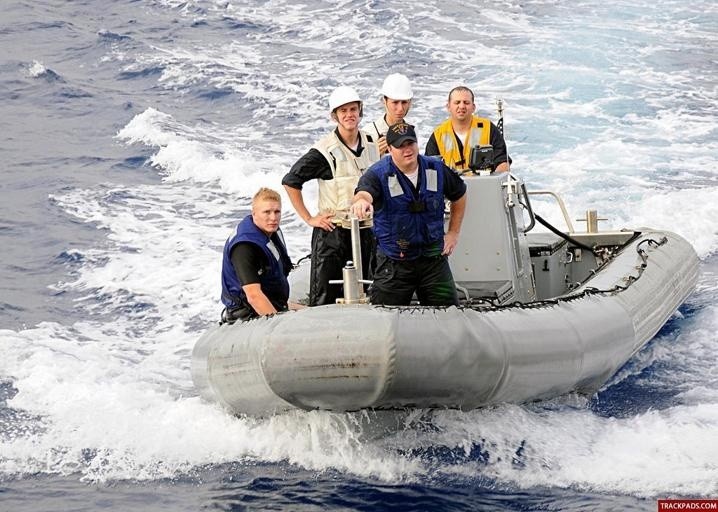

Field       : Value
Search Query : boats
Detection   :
[188,226,705,439]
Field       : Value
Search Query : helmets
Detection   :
[327,86,364,113]
[379,72,415,100]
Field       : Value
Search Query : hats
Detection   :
[384,122,419,149]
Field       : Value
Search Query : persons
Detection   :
[219,187,310,322]
[359,72,419,158]
[282,84,383,304]
[424,86,513,174]
[349,122,466,307]
